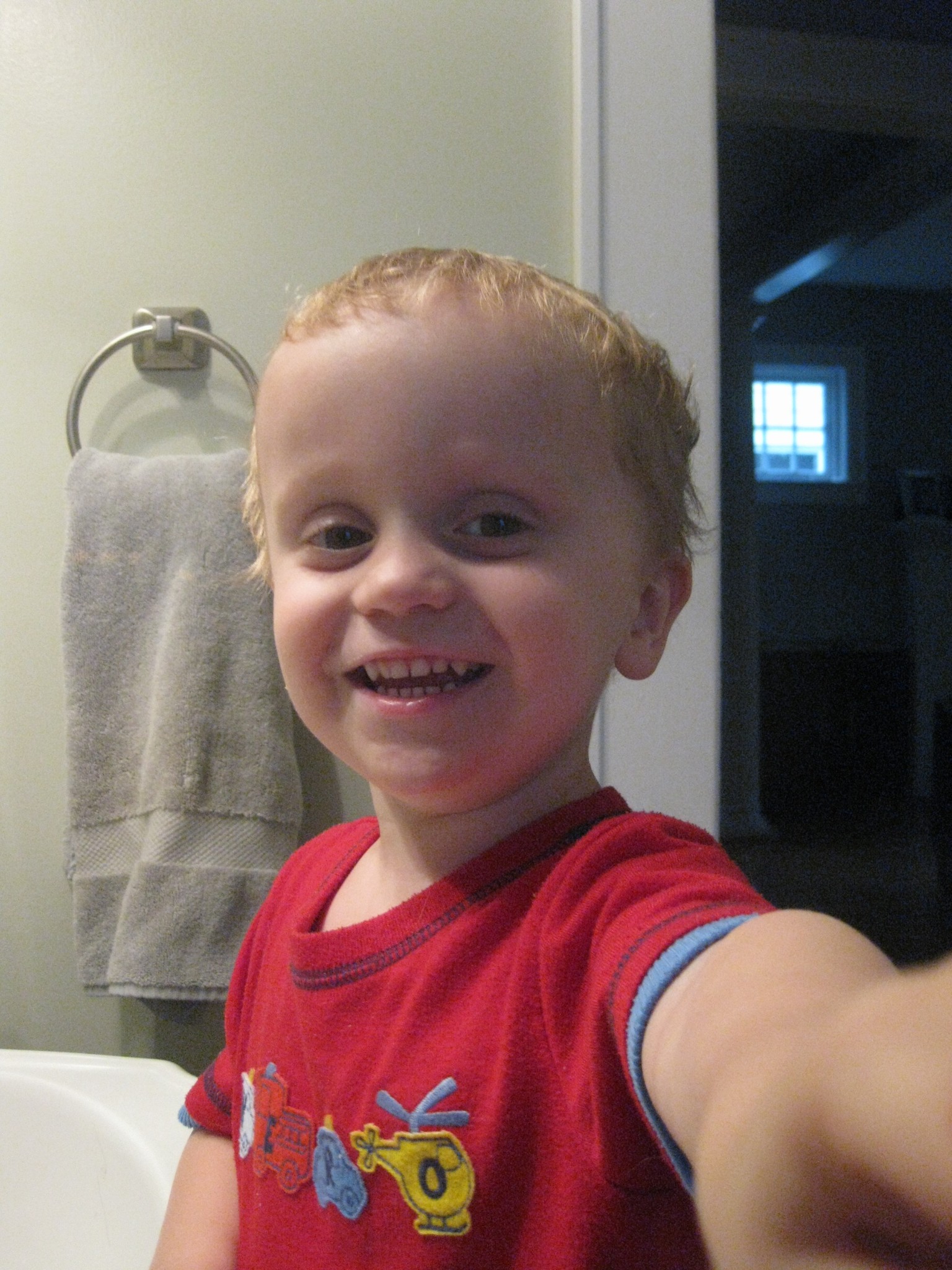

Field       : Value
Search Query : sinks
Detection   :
[3,1047,210,1264]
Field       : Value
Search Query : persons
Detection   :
[147,247,952,1270]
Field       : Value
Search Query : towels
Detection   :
[59,446,312,1008]
